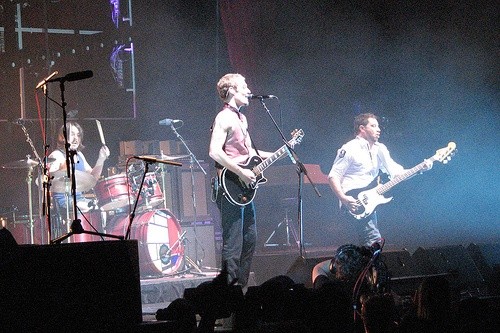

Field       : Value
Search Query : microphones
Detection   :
[49,70,93,82]
[135,155,183,166]
[159,118,179,125]
[35,71,58,89]
[248,94,274,99]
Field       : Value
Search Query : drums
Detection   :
[49,209,107,244]
[106,208,185,275]
[129,172,164,209]
[92,173,137,211]
[1,215,50,245]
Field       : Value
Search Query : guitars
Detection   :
[220,128,306,206]
[344,140,459,220]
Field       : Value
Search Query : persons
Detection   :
[312,244,500,333]
[36,121,110,238]
[327,112,433,248]
[209,74,294,289]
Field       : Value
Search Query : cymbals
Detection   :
[156,153,191,161]
[0,157,56,168]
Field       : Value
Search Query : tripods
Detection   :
[168,125,224,274]
[48,82,124,244]
[266,198,299,249]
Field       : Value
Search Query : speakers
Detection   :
[286,256,334,288]
[164,163,210,220]
[409,246,484,290]
[178,220,217,272]
[468,242,500,284]
[376,249,421,294]
